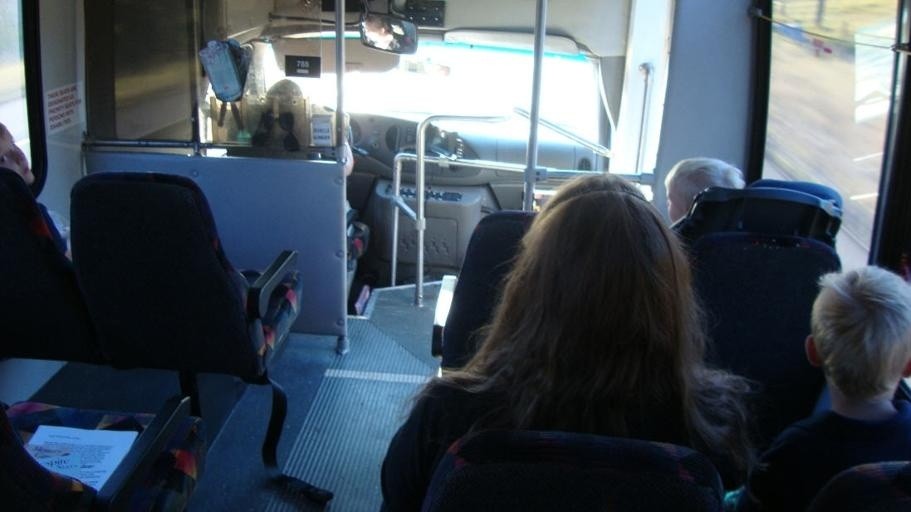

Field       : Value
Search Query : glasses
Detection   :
[276,109,301,154]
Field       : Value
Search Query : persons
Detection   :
[664,156,747,227]
[747,264,911,512]
[216,78,355,178]
[362,18,414,52]
[376,167,746,511]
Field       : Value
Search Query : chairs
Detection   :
[0,384,204,512]
[70,172,310,418]
[228,98,369,273]
[0,167,75,361]
